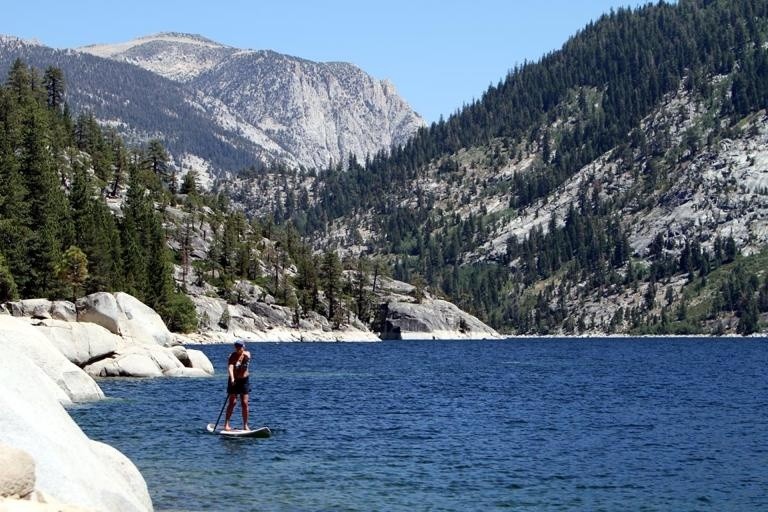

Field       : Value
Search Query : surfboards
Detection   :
[205,423,270,437]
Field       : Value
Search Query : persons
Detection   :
[224,339,251,431]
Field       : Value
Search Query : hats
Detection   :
[235,341,245,347]
